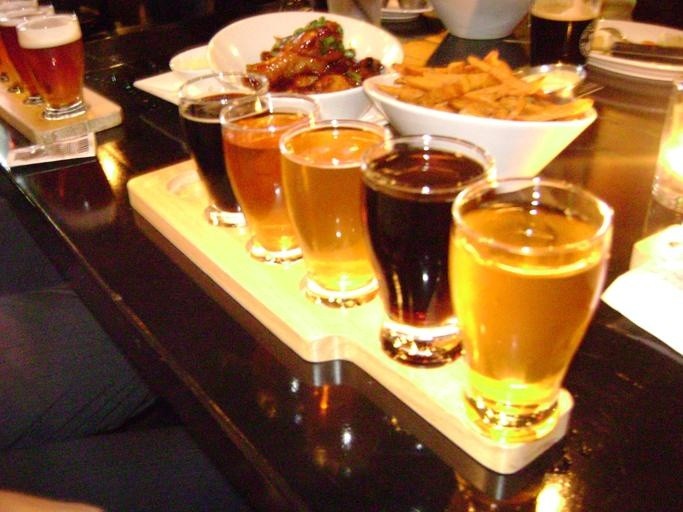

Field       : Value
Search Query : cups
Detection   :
[0,0,86,121]
[362,132,495,368]
[169,45,209,82]
[527,0,601,70]
[650,80,683,215]
[215,93,319,265]
[176,71,271,228]
[445,170,615,446]
[277,118,395,306]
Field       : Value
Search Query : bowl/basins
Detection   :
[208,12,405,124]
[362,71,600,185]
[431,0,530,40]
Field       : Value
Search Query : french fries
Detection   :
[376,48,595,122]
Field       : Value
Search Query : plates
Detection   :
[380,5,434,23]
[584,21,683,85]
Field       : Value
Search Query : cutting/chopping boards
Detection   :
[126,155,573,479]
[0,78,125,146]
